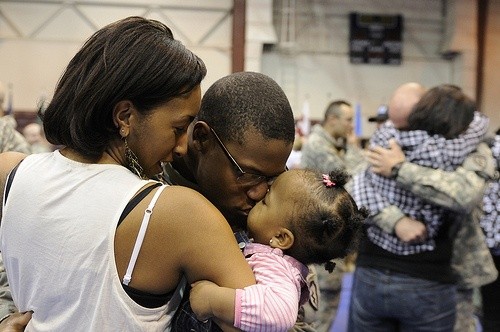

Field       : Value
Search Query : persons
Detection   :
[300,100,365,332]
[0,71,298,332]
[0,90,53,154]
[186,167,370,332]
[0,13,258,332]
[344,81,498,332]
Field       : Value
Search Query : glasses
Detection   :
[210,128,290,187]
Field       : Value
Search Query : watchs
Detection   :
[390,161,405,179]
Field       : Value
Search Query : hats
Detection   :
[368,105,389,122]
